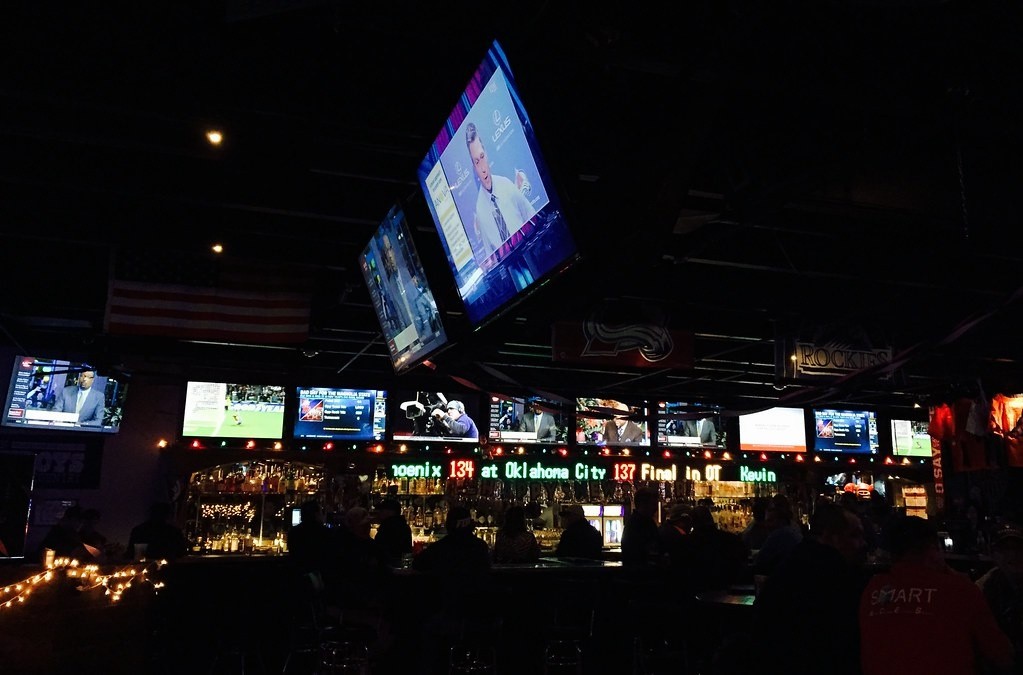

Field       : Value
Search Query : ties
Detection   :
[618,428,623,440]
[697,422,702,436]
[491,195,511,240]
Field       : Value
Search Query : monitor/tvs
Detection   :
[888,418,933,460]
[293,384,389,442]
[393,388,481,447]
[0,352,135,437]
[416,38,582,333]
[573,394,653,450]
[654,396,728,451]
[177,379,288,442]
[360,201,461,377]
[736,405,809,454]
[812,405,883,456]
[487,392,571,447]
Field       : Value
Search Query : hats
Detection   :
[989,521,1023,546]
[558,505,584,517]
[447,400,465,415]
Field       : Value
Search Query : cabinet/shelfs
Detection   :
[186,490,446,559]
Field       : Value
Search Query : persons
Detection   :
[494,507,540,563]
[431,401,478,438]
[465,123,535,254]
[35,509,185,561]
[255,495,338,553]
[382,234,433,342]
[52,370,105,425]
[937,501,998,560]
[860,516,1013,675]
[750,504,872,675]
[225,384,285,425]
[375,500,412,568]
[684,411,716,445]
[413,506,490,628]
[556,506,601,559]
[977,521,1023,675]
[622,487,745,591]
[313,508,391,656]
[520,407,556,441]
[605,404,642,442]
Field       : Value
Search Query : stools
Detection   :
[144,567,758,675]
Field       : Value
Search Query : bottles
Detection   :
[184,461,931,557]
